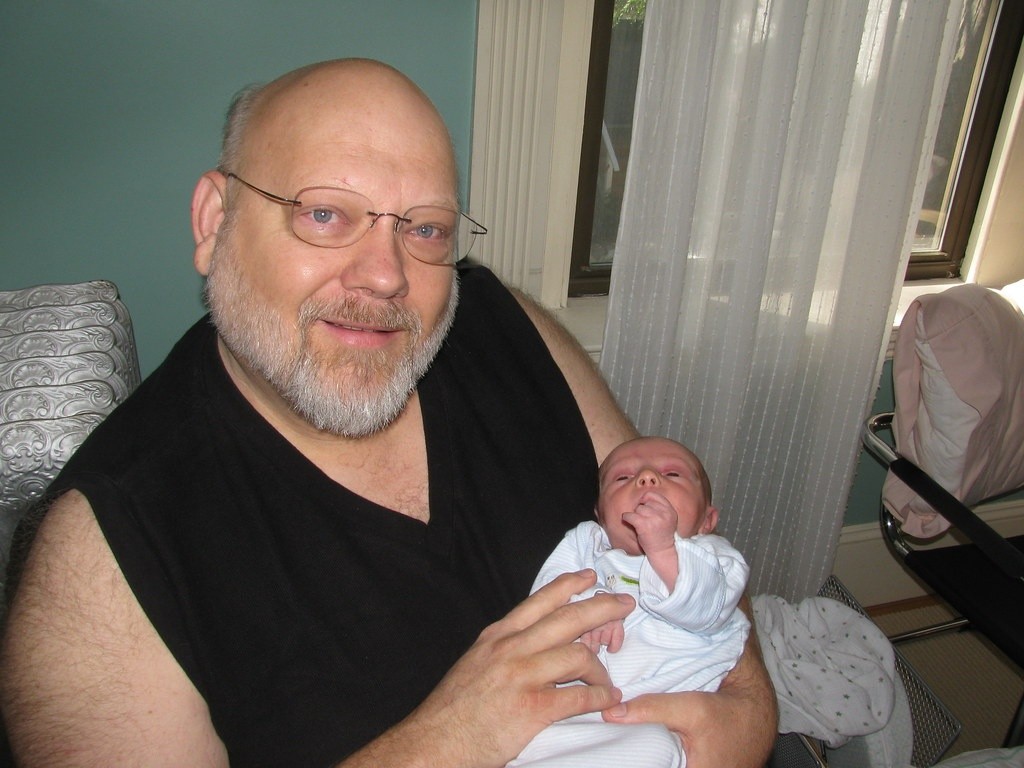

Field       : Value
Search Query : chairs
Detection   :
[856,410,1022,768]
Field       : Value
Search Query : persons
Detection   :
[0,57,780,767]
[507,435,752,768]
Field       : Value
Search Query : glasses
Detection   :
[216,166,488,267]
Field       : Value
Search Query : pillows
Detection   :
[880,288,1024,545]
